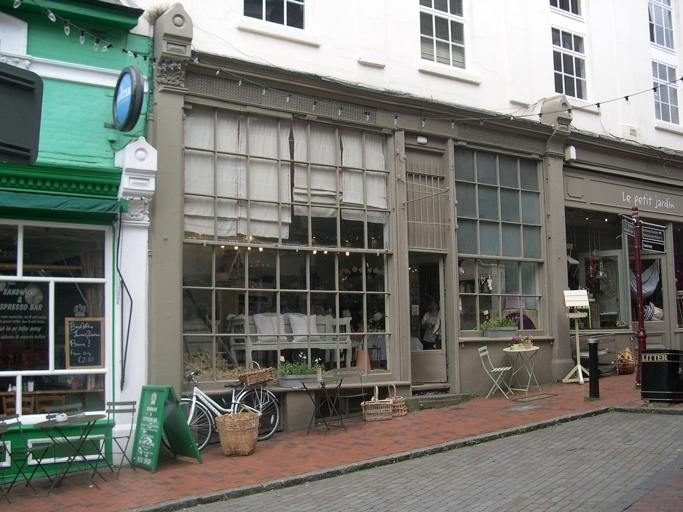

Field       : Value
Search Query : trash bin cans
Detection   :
[640,349,683,403]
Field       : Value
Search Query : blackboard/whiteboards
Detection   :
[0,279,48,348]
[64,317,104,369]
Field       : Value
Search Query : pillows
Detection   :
[253,313,288,344]
[287,314,322,342]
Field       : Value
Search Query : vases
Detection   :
[511,344,520,350]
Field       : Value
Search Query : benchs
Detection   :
[226,313,353,369]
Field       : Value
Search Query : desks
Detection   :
[503,346,541,398]
[299,377,348,435]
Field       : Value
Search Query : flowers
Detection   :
[512,335,522,344]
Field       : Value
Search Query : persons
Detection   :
[420,301,439,350]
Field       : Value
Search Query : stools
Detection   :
[368,347,382,369]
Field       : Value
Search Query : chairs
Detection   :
[334,367,368,429]
[478,346,514,400]
[0,393,136,505]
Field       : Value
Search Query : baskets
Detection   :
[613,348,637,376]
[237,359,276,387]
[385,382,408,417]
[215,411,260,457]
[358,385,393,421]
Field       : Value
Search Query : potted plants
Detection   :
[524,333,534,349]
[277,363,317,387]
[481,316,519,338]
